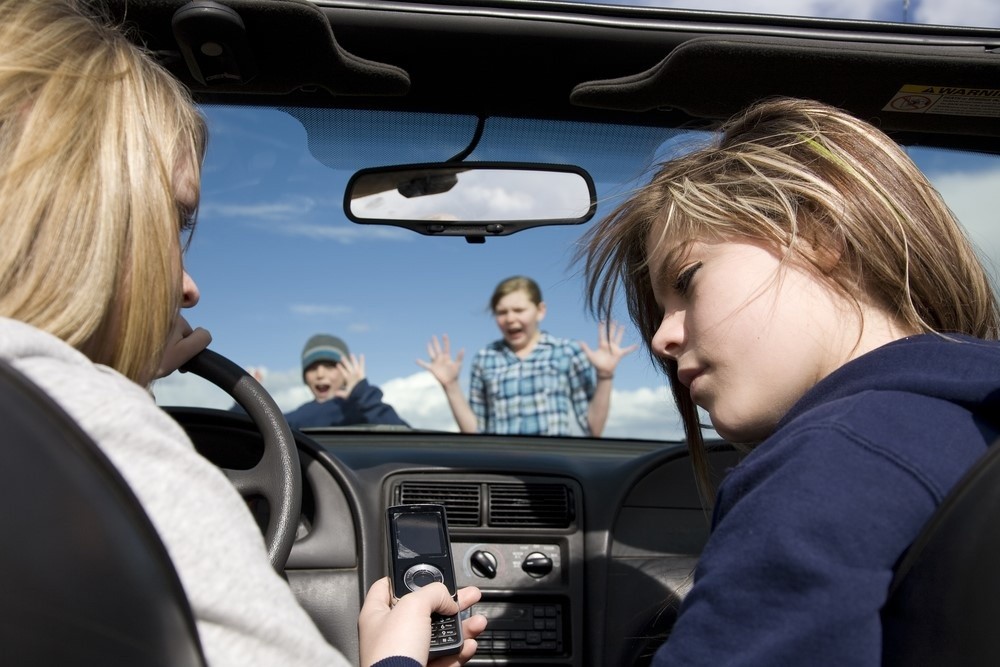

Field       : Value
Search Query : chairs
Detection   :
[0,355,208,667]
[880,440,1000,667]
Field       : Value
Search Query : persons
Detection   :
[359,101,1000,667]
[0,0,353,667]
[415,276,636,437]
[226,334,411,427]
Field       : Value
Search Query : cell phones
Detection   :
[386,502,464,656]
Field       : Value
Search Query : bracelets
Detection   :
[596,374,614,379]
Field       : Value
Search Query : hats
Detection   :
[301,334,350,384]
